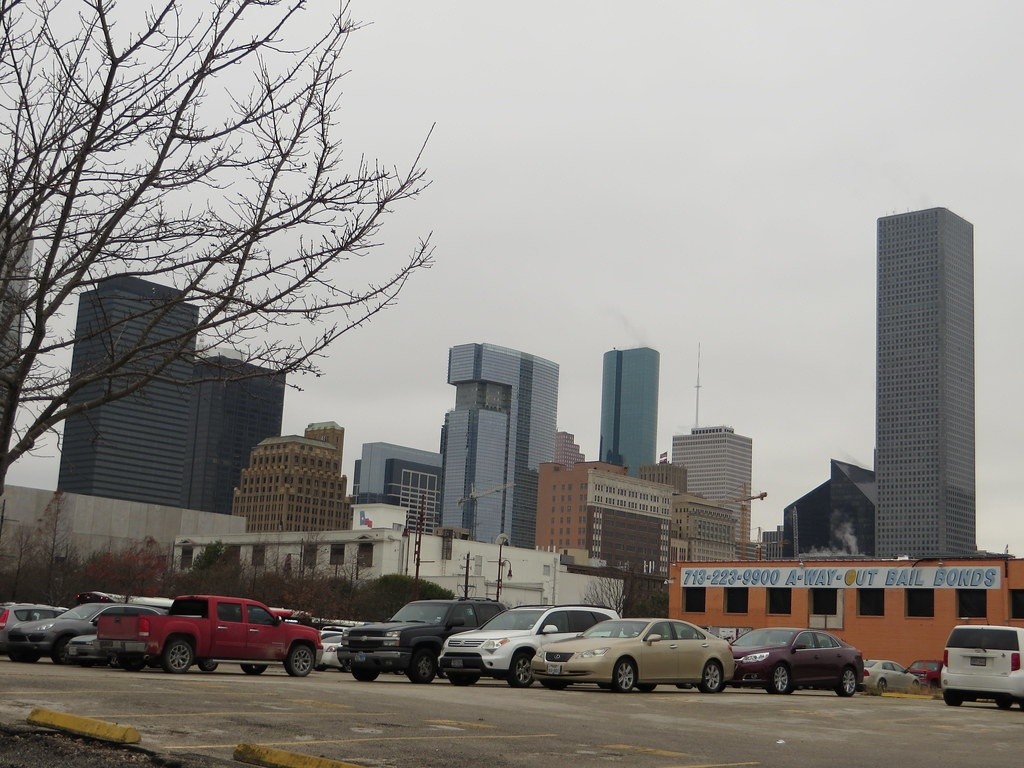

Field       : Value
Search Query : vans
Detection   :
[940,625,1023,712]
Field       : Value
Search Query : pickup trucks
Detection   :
[97,595,325,678]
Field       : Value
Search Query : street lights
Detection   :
[496,536,513,602]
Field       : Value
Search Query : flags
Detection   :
[660,452,667,458]
[365,519,372,529]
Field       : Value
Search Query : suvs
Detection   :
[438,604,625,688]
[337,598,515,684]
[0,602,81,664]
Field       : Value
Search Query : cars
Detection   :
[528,618,736,695]
[906,659,946,691]
[730,626,865,698]
[5,602,170,664]
[858,659,922,696]
[250,605,349,673]
[64,634,121,669]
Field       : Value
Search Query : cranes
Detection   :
[716,484,767,561]
[747,527,792,562]
[458,480,517,541]
